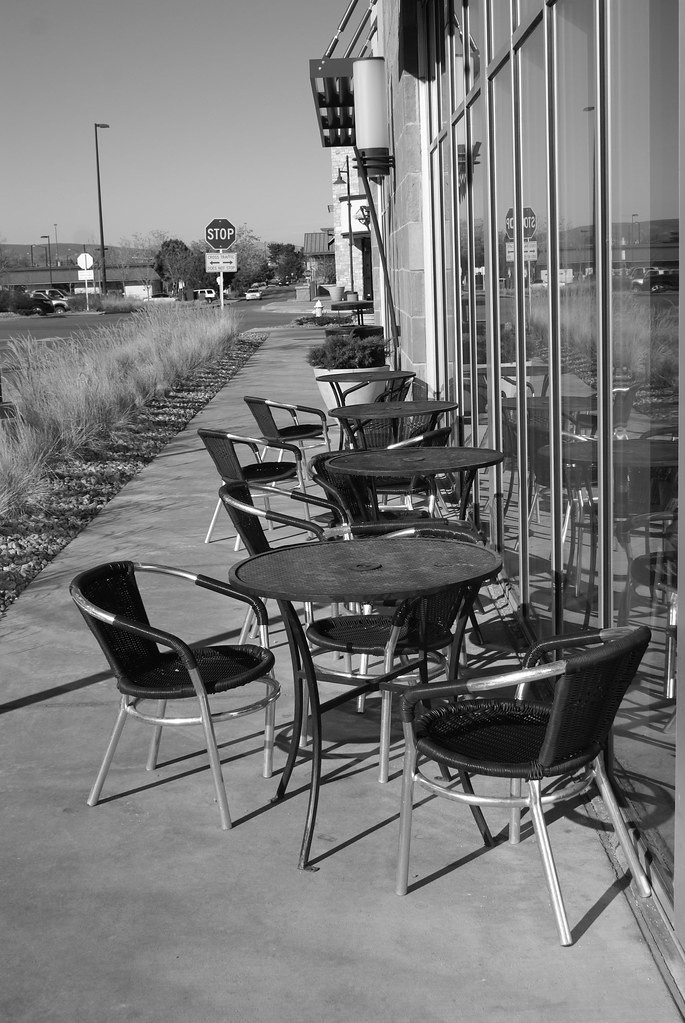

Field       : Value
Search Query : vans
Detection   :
[193,288,217,304]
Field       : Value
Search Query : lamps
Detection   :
[351,56,397,183]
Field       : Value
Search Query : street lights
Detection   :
[95,123,110,296]
[41,236,52,289]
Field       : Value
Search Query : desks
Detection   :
[326,445,505,531]
[227,535,506,876]
[331,301,374,328]
[327,399,458,453]
[316,370,418,452]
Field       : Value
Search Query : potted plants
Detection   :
[305,335,394,427]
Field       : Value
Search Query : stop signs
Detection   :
[205,218,236,250]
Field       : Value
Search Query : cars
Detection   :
[29,289,73,314]
[143,293,179,302]
[245,287,262,301]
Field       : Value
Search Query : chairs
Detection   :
[395,623,655,948]
[68,557,282,834]
[195,427,312,531]
[378,425,453,519]
[243,396,332,489]
[217,478,356,649]
[303,521,489,714]
[507,336,677,605]
